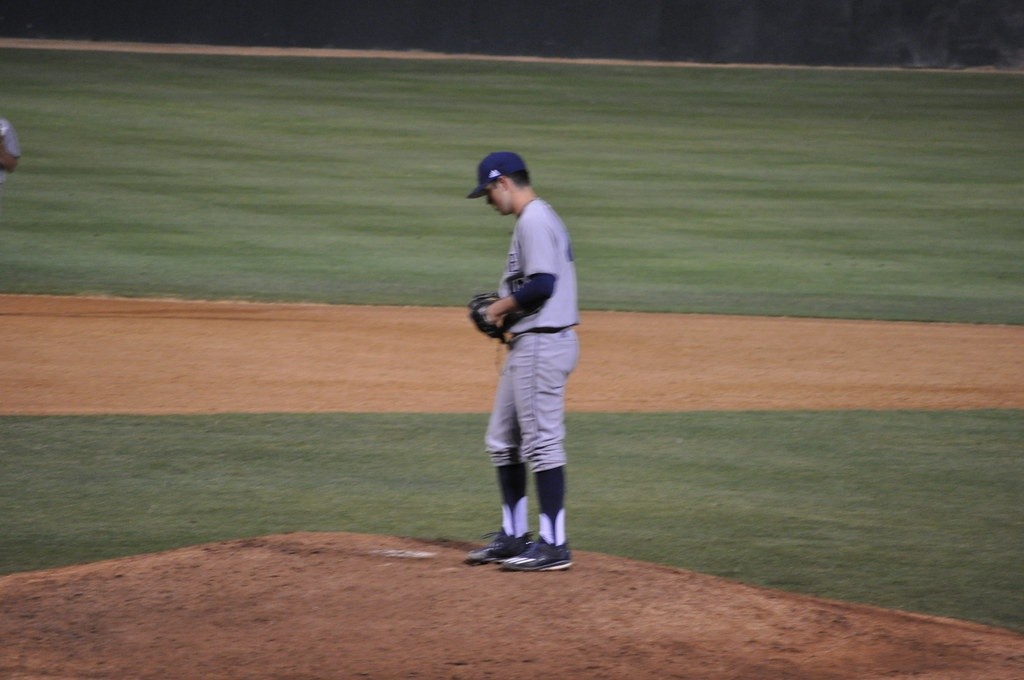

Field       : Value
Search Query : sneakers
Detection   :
[503,537,573,571]
[463,527,534,566]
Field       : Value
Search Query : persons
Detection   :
[465,151,584,574]
[0,116,22,184]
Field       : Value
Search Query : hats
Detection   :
[465,151,527,199]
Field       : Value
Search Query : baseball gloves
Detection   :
[466,292,510,338]
